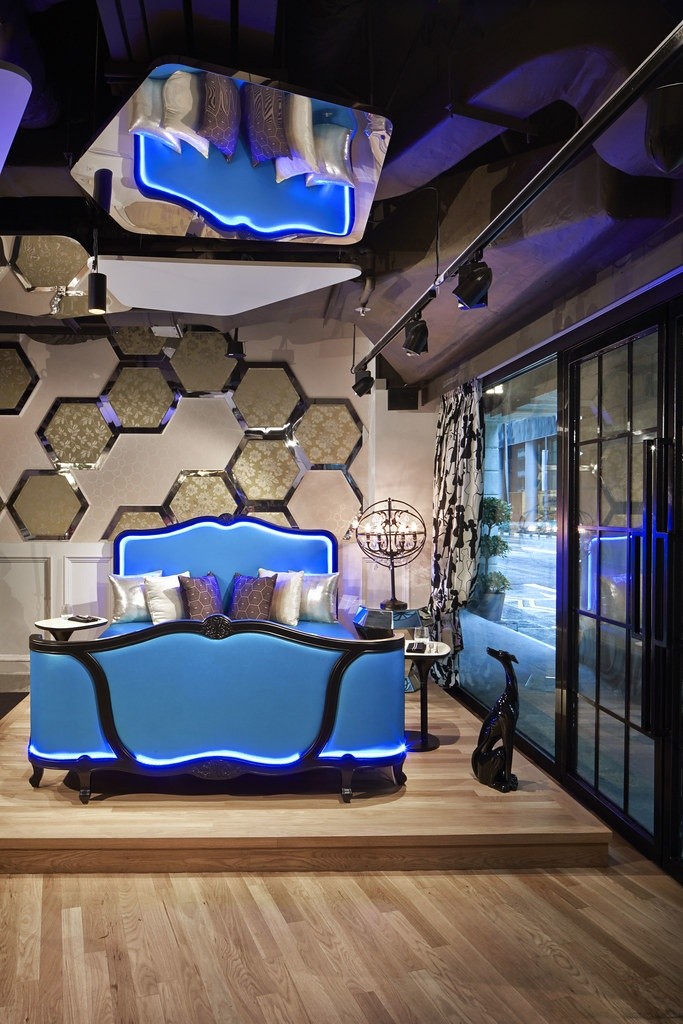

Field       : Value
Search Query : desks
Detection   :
[34,614,108,642]
[405,638,451,752]
[352,605,433,692]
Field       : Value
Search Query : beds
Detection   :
[26,513,407,803]
[134,63,357,241]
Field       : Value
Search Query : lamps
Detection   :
[87,261,107,315]
[644,64,683,173]
[226,341,246,358]
[353,364,375,397]
[355,498,427,610]
[452,249,492,310]
[403,312,429,357]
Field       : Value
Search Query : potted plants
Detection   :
[468,496,514,621]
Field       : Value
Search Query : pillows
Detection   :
[197,71,241,164]
[238,82,295,167]
[128,77,183,155]
[276,94,321,184]
[178,570,224,620]
[257,568,304,626]
[227,572,279,620]
[107,570,163,623]
[288,569,340,625]
[144,571,191,626]
[160,71,211,159]
[306,123,355,189]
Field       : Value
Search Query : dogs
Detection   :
[471,647,519,793]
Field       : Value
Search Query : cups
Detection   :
[414,627,429,644]
